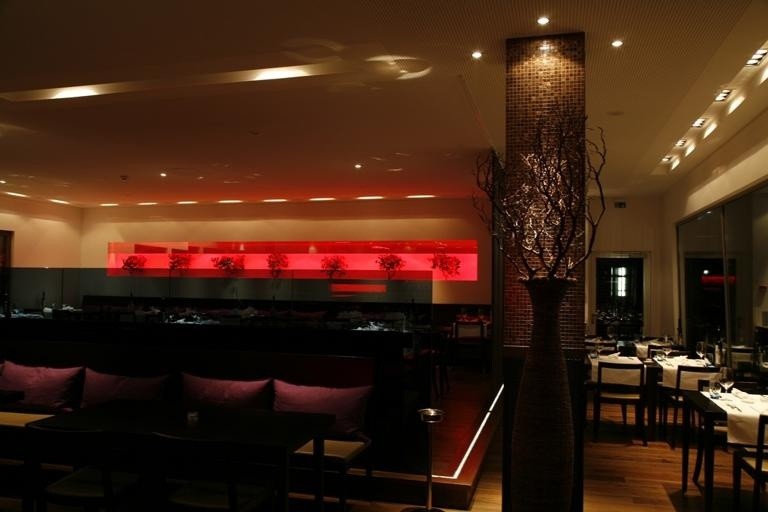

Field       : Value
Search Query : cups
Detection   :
[708,379,721,395]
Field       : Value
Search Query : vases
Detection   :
[508,277,576,511]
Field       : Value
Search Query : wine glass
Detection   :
[718,366,735,396]
[661,342,673,359]
[695,341,705,360]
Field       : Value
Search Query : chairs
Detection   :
[584,322,768,512]
